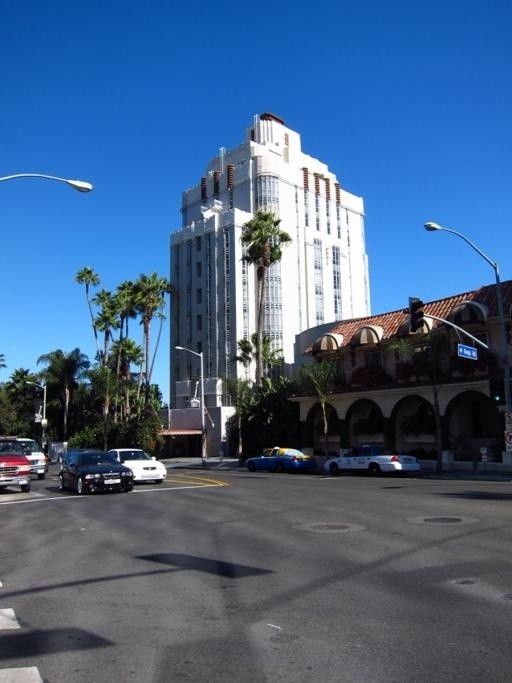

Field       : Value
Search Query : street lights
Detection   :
[424,222,512,464]
[26,382,46,448]
[0,174,93,192]
[175,346,207,467]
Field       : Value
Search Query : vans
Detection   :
[0,436,47,492]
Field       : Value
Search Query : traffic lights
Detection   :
[488,367,506,405]
[409,297,424,334]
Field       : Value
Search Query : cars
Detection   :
[58,449,166,495]
[324,445,421,476]
[245,447,317,472]
